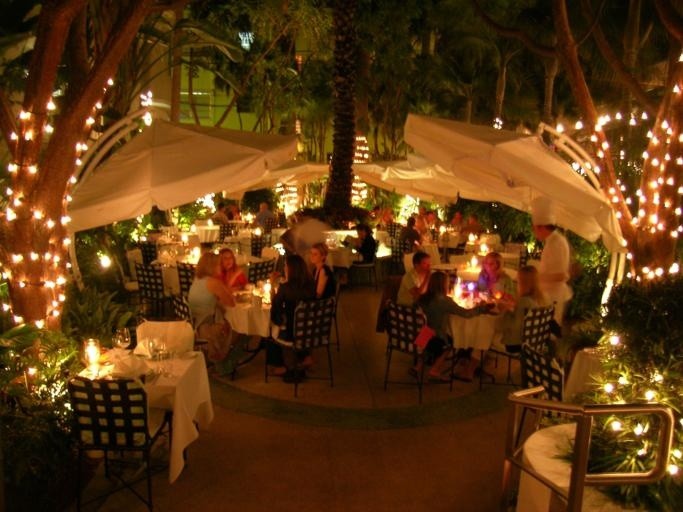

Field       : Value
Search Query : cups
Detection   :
[83,339,103,376]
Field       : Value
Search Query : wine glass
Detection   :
[450,277,504,311]
[244,280,278,304]
[146,337,173,377]
[326,234,341,248]
[112,327,132,361]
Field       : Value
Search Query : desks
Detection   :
[563,345,615,400]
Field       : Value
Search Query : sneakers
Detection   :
[408,367,474,385]
[282,362,314,384]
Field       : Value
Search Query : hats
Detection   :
[529,196,557,225]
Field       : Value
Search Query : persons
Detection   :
[531,198,574,342]
[188,253,264,364]
[385,202,545,382]
[266,253,317,374]
[310,242,334,301]
[218,248,249,295]
[212,202,276,232]
[347,223,376,263]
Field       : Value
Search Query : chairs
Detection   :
[72,205,379,399]
[377,223,565,450]
[67,375,172,511]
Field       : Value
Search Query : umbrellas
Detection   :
[221,158,331,195]
[59,104,298,295]
[403,113,630,326]
[352,152,502,209]
